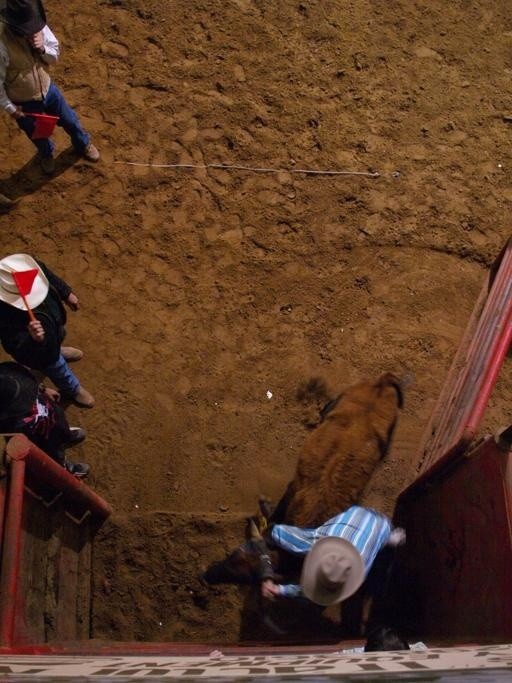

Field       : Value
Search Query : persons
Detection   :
[0,0,101,175]
[1,253,98,409]
[263,505,408,608]
[0,359,93,480]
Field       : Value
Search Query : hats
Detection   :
[1,1,47,35]
[299,536,364,606]
[1,362,37,419]
[0,254,50,311]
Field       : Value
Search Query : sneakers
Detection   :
[65,463,89,478]
[74,388,96,408]
[41,161,55,174]
[60,347,83,361]
[68,427,85,442]
[85,145,100,161]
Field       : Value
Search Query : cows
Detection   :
[251,369,407,568]
